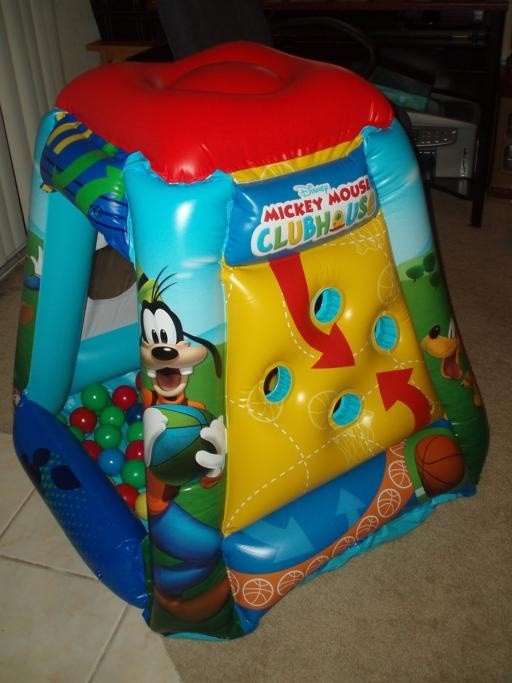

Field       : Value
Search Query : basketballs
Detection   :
[415,435,464,496]
[150,405,218,487]
